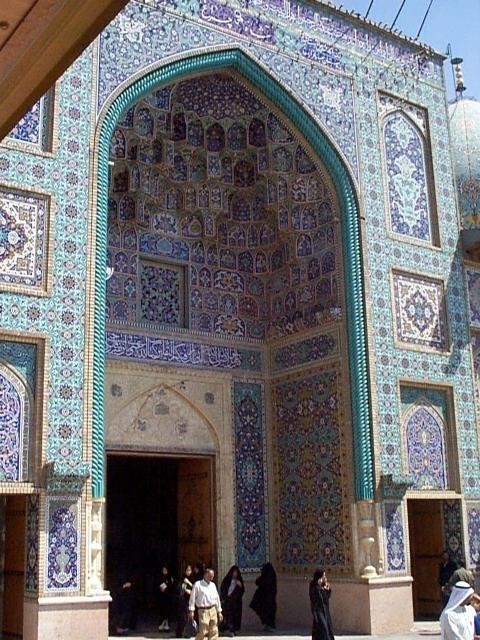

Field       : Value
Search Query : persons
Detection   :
[309,568,335,640]
[110,565,245,640]
[439,551,480,640]
[250,563,277,632]
